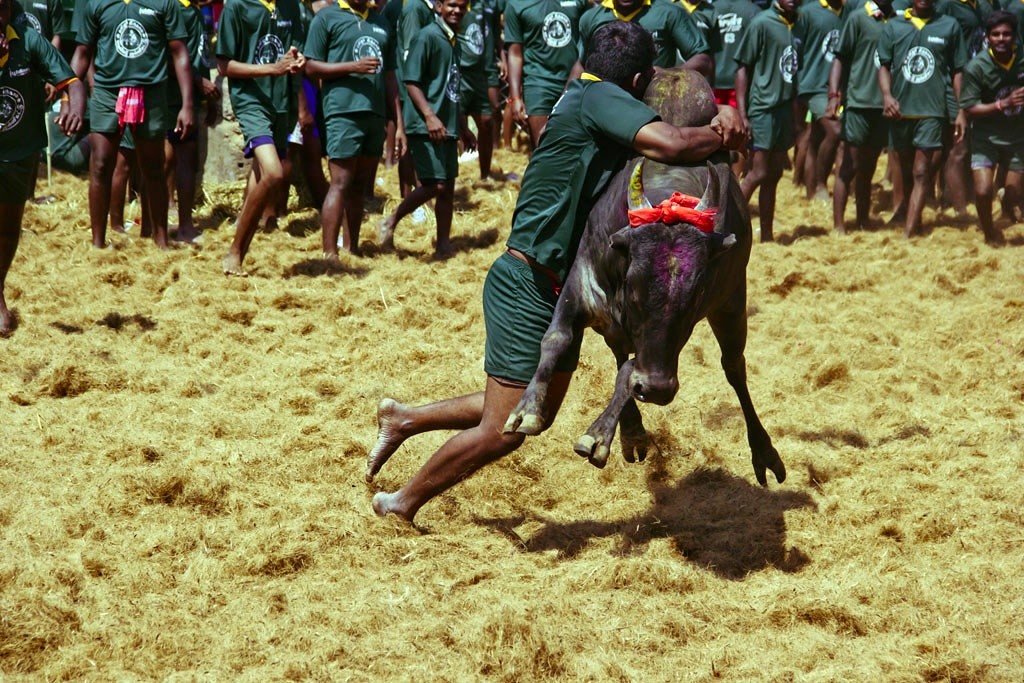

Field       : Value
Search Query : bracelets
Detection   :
[828,92,838,100]
[996,100,1002,112]
[510,96,521,103]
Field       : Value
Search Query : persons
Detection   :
[366,21,749,523]
[0,0,1024,332]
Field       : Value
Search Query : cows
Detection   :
[504,69,788,489]
